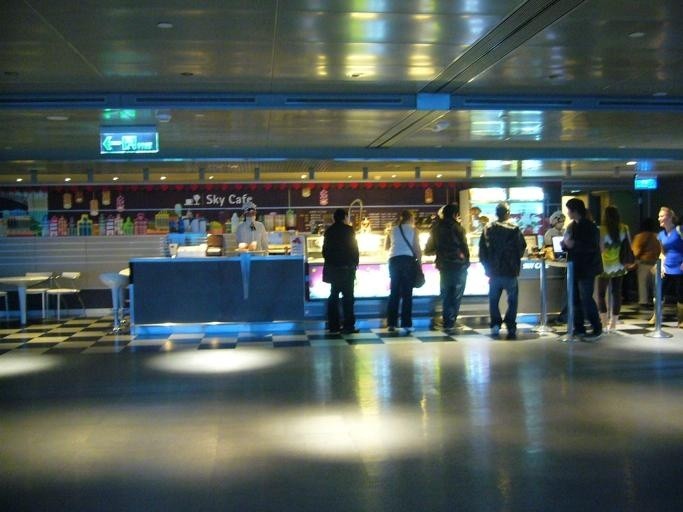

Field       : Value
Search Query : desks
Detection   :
[1,277,48,328]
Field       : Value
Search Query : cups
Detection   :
[168,244,178,258]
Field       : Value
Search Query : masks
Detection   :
[556,223,564,230]
[246,216,256,223]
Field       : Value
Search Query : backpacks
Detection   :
[492,232,521,276]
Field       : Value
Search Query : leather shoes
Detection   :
[329,327,342,332]
[343,329,360,333]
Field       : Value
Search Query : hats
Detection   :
[549,212,565,225]
[242,202,256,214]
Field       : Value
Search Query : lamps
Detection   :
[320,189,329,206]
[302,188,312,200]
[90,190,102,217]
[75,191,84,203]
[425,187,434,203]
[63,189,74,209]
[117,193,127,213]
[102,190,112,206]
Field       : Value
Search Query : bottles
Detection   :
[2,212,206,237]
[224,210,297,234]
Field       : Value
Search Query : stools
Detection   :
[98,273,129,335]
[1,291,9,322]
[25,271,52,319]
[119,268,131,327]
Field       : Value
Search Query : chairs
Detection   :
[47,272,86,322]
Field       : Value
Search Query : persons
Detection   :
[323,207,359,335]
[553,208,596,325]
[595,206,633,334]
[646,205,683,330]
[559,198,604,339]
[235,202,269,254]
[382,208,423,335]
[478,202,529,340]
[430,204,470,331]
[629,218,661,305]
[423,204,448,328]
[543,211,565,246]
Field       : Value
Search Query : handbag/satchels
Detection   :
[413,260,425,287]
[425,223,440,256]
[620,225,634,263]
